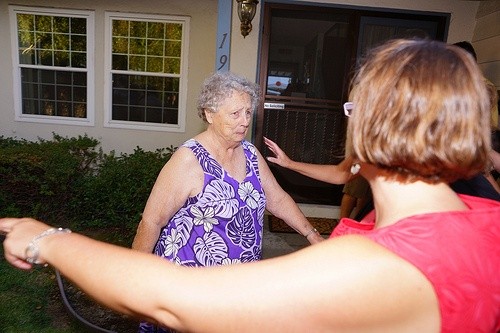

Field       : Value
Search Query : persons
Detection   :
[132,72,326,333]
[452,39,499,201]
[0,39,500,333]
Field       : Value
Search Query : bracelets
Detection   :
[26,227,71,266]
[306,228,317,239]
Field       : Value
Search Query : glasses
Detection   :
[343,102,355,117]
